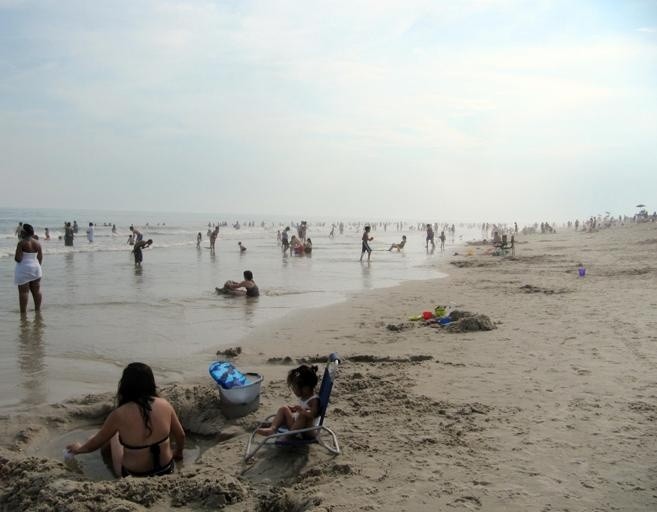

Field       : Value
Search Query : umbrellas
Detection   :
[636,203,647,209]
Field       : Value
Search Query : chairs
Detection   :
[241,351,341,462]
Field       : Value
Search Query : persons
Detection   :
[215,270,260,297]
[14,224,42,313]
[14,220,141,246]
[258,364,319,435]
[132,239,153,265]
[65,360,184,478]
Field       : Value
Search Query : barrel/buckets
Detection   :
[423,312,432,320]
[578,268,586,276]
[219,372,264,419]
